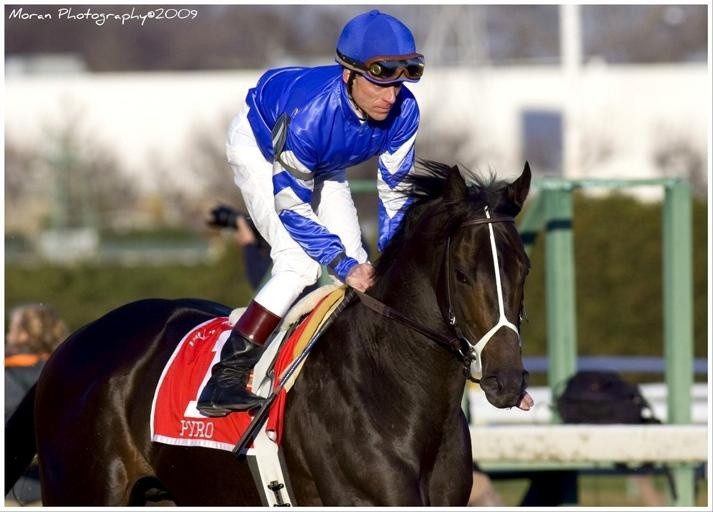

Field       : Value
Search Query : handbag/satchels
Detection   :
[559,371,641,423]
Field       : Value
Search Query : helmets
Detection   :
[334,10,420,85]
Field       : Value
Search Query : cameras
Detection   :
[205,200,258,237]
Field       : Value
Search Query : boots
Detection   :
[197,299,284,416]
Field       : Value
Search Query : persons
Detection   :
[5,302,70,504]
[223,215,274,292]
[197,8,421,419]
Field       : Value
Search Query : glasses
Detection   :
[336,47,425,83]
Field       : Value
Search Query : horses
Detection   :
[4,157,534,507]
